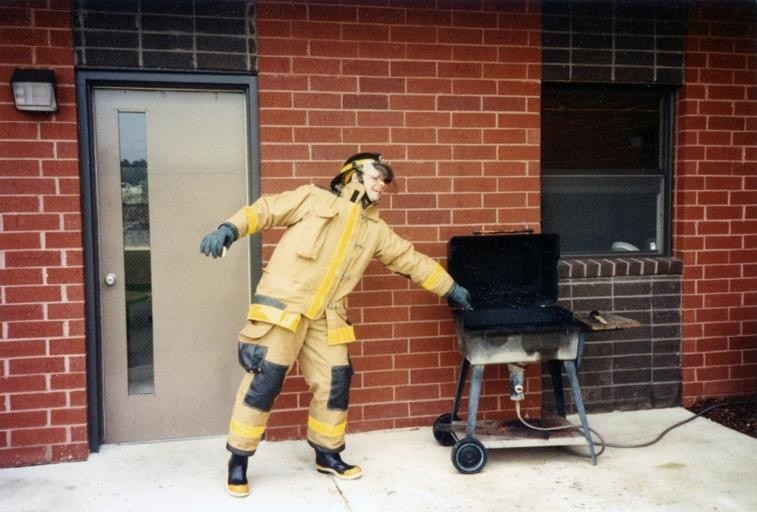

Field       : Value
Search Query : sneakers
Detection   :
[314,449,363,481]
[227,452,254,496]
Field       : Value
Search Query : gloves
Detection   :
[199,225,238,259]
[449,283,473,311]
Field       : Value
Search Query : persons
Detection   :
[199,152,476,498]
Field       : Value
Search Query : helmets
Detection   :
[329,151,395,191]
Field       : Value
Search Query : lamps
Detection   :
[10,69,59,115]
